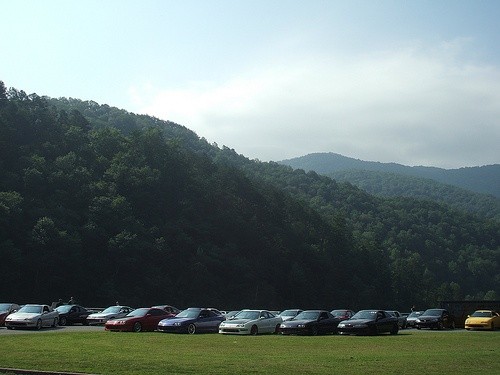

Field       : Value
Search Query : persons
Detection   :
[56,299,63,308]
[67,296,77,305]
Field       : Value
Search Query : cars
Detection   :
[279,310,341,336]
[385,311,411,329]
[337,309,400,336]
[104,307,177,332]
[151,305,183,315]
[0,302,21,329]
[85,305,136,326]
[464,309,500,331]
[405,310,425,329]
[414,308,456,330]
[330,309,355,322]
[277,309,304,322]
[218,309,283,336]
[219,309,281,321]
[157,307,226,335]
[54,304,94,327]
[5,304,59,331]
